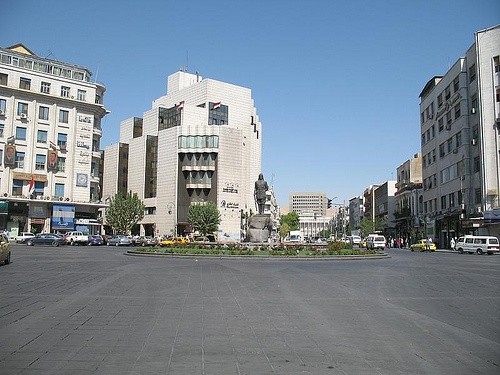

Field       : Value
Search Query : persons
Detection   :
[426,237,431,253]
[433,236,437,246]
[132,234,139,246]
[3,229,9,238]
[450,237,457,250]
[255,174,268,214]
[387,235,411,249]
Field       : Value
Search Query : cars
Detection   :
[359,238,366,248]
[304,233,361,246]
[89,235,104,246]
[0,233,11,264]
[15,232,36,244]
[410,238,437,252]
[101,233,219,248]
[25,232,65,247]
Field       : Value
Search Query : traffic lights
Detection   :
[327,199,332,209]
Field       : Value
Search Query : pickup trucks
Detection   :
[65,231,89,246]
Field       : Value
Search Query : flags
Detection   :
[29,177,35,193]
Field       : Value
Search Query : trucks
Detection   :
[289,230,304,243]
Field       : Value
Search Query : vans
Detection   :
[365,235,387,251]
[455,235,500,255]
